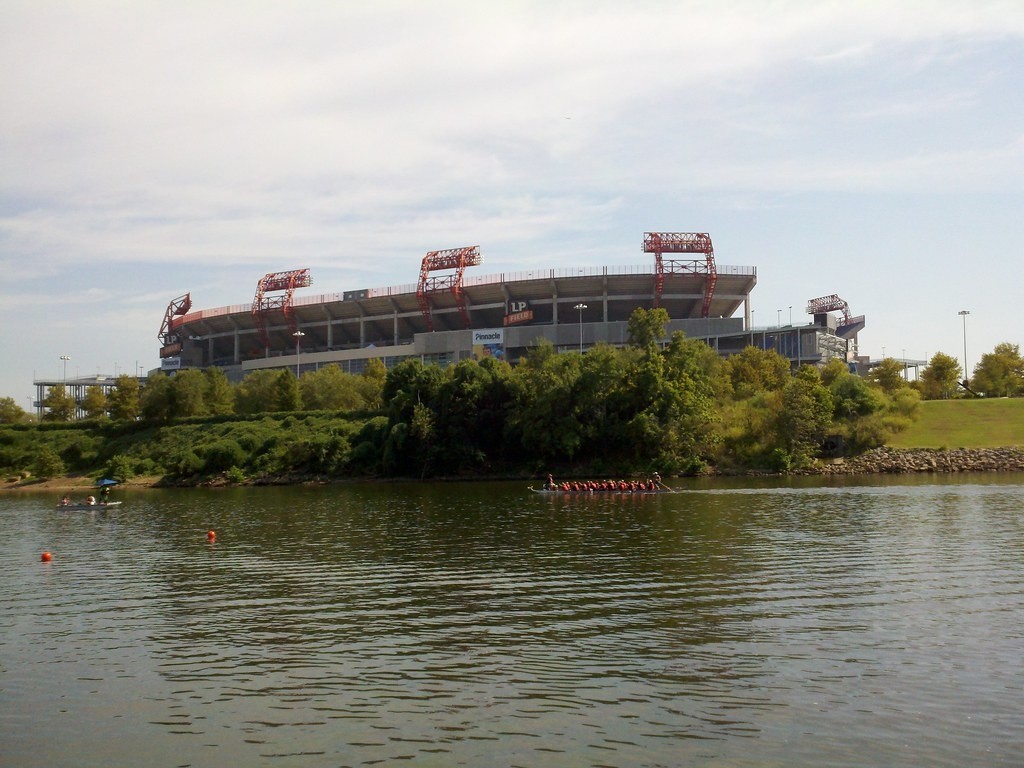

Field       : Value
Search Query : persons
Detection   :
[546,472,661,490]
[87,495,96,505]
[62,496,68,505]
[98,484,109,505]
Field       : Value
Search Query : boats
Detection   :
[527,485,690,496]
[56,502,122,509]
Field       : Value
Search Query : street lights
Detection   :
[293,331,306,378]
[574,304,588,355]
[60,356,70,399]
[957,309,971,380]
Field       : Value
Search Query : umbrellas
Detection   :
[95,479,117,485]
